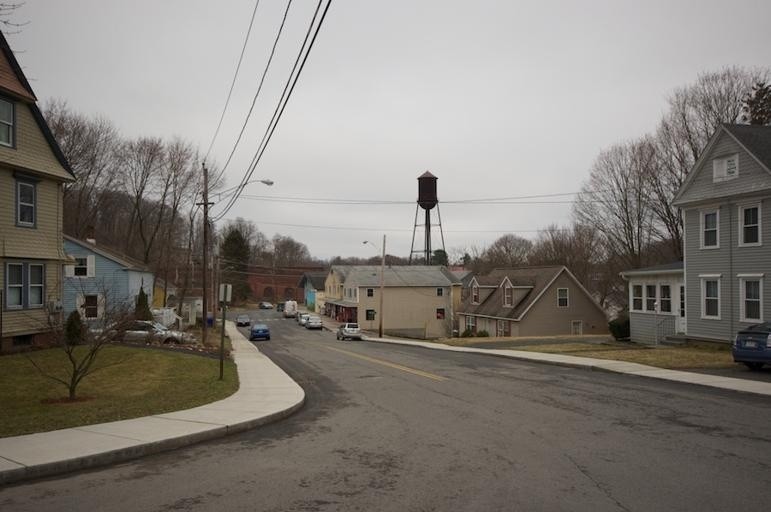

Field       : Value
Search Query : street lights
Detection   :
[199,178,273,336]
[363,240,386,338]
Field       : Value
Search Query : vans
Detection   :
[285,301,297,318]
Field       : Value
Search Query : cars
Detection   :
[91,316,196,344]
[337,323,361,341]
[235,314,250,326]
[248,324,270,341]
[296,310,322,330]
[732,321,771,371]
[259,302,273,309]
[277,302,285,312]
